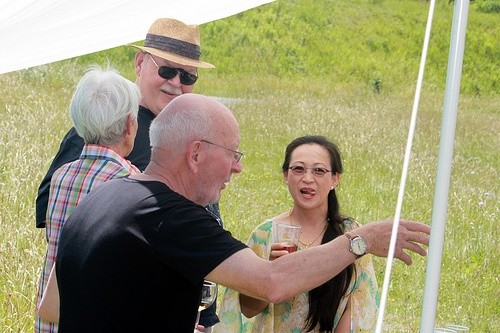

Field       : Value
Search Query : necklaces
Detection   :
[288,213,327,249]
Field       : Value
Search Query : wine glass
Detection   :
[195,280,218,328]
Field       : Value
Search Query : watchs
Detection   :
[345,231,367,261]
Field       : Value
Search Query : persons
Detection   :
[240,136,381,333]
[39,94,431,333]
[36,18,222,327]
[34,64,143,333]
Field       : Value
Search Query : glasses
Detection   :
[144,52,199,85]
[200,139,243,163]
[288,165,333,177]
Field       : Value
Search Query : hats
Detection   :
[122,18,216,68]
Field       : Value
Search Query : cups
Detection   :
[277,222,302,253]
[434,324,469,333]
